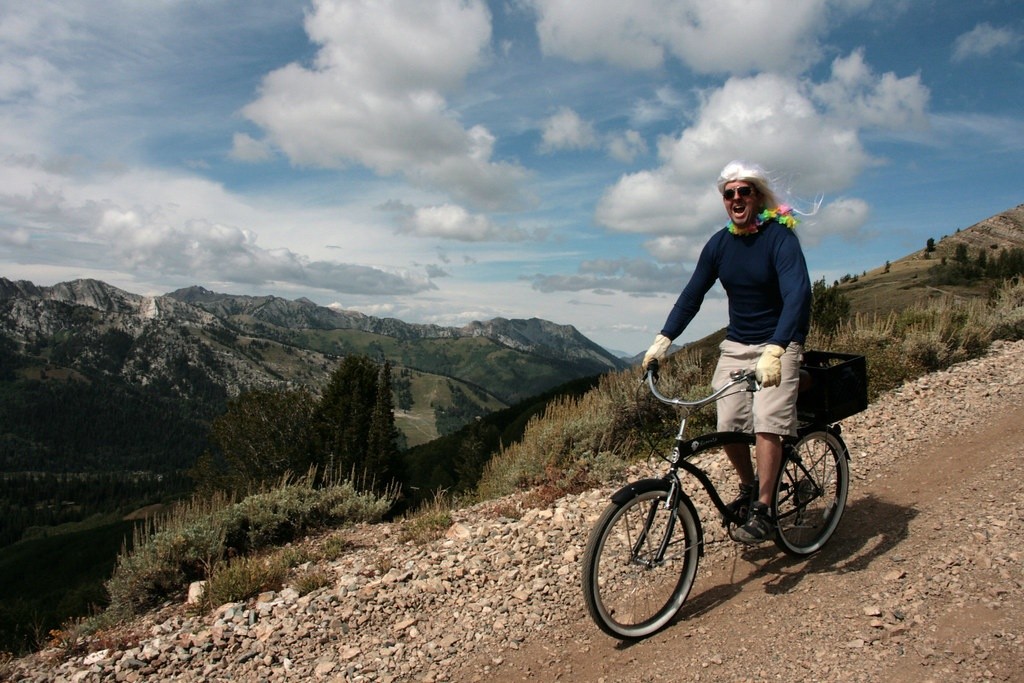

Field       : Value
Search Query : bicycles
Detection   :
[580,355,853,639]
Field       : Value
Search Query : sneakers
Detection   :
[735,501,778,543]
[724,475,759,520]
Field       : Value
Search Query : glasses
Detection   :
[722,186,758,201]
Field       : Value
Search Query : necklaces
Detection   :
[725,203,802,237]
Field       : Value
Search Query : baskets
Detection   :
[795,349,869,426]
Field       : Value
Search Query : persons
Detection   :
[639,159,818,545]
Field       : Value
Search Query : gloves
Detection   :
[754,344,785,388]
[642,332,672,373]
